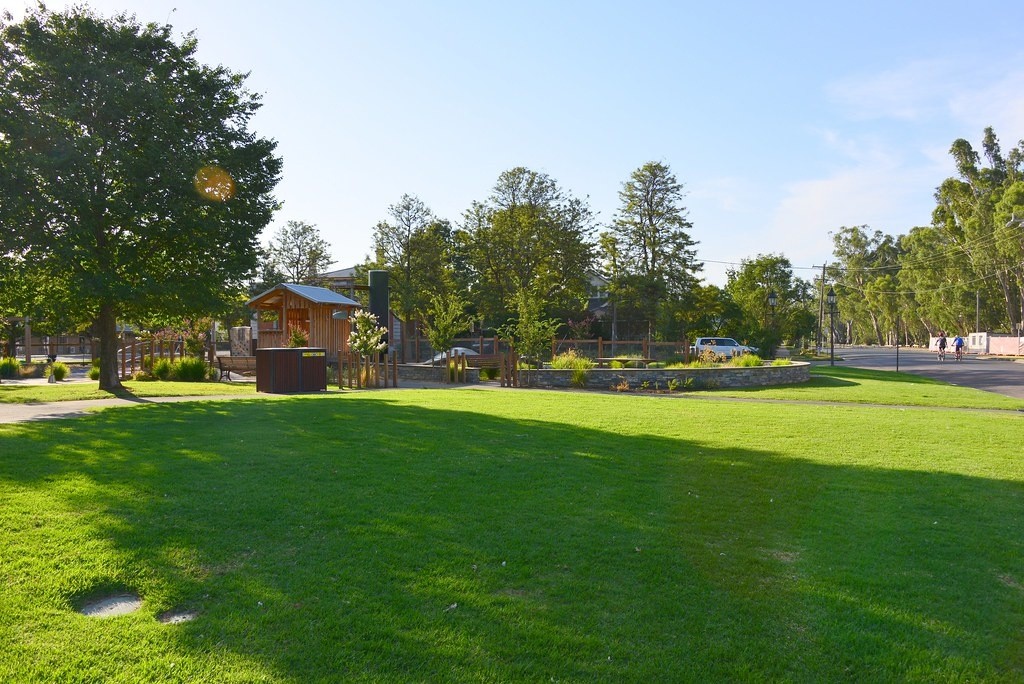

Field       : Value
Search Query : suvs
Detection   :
[690,337,752,361]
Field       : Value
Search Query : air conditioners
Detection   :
[69,347,75,353]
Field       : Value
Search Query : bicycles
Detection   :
[951,344,965,360]
[936,344,947,361]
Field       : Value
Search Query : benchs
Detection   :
[466,353,504,369]
[217,356,256,382]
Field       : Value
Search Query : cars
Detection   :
[422,346,481,366]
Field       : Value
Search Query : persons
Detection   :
[951,335,964,359]
[935,333,947,359]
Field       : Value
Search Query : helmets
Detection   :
[939,332,943,336]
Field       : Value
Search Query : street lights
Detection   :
[824,285,840,366]
[767,289,778,360]
